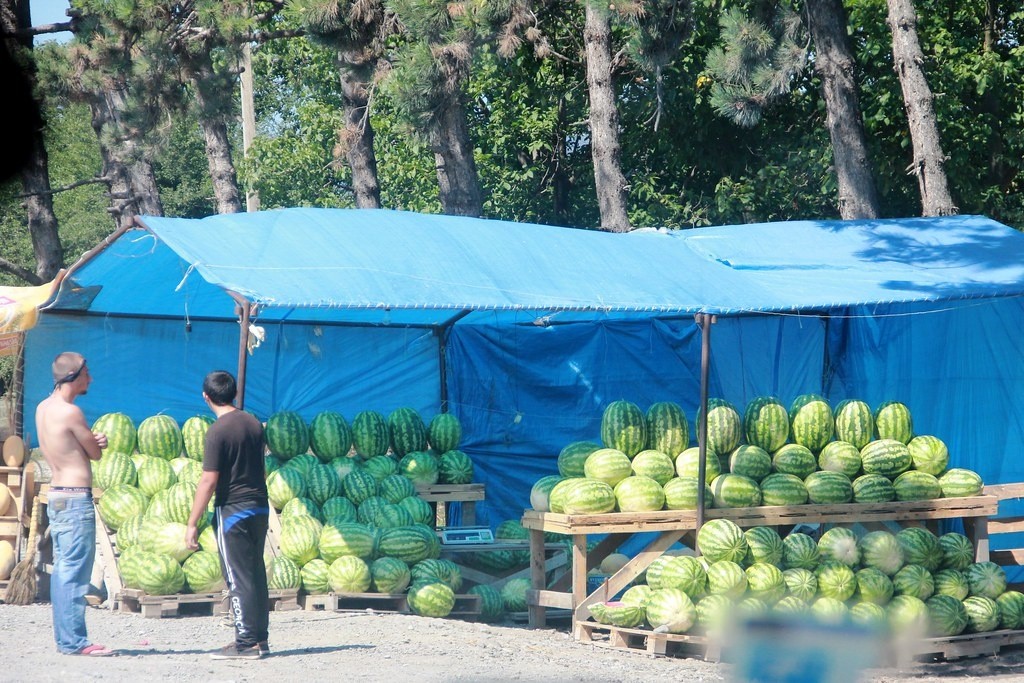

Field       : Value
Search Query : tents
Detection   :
[22,206,1024,587]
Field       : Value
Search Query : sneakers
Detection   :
[210,639,261,658]
[259,642,272,655]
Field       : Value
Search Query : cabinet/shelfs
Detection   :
[0,464,37,584]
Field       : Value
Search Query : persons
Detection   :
[184,370,271,660]
[35,350,112,657]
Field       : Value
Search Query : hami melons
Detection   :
[0,435,25,580]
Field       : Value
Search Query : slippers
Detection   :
[78,646,112,656]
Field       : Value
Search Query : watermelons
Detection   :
[588,519,1024,636]
[530,396,985,517]
[89,405,573,623]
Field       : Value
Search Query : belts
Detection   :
[50,485,93,493]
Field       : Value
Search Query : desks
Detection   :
[435,538,571,622]
[519,494,998,657]
[415,483,485,530]
